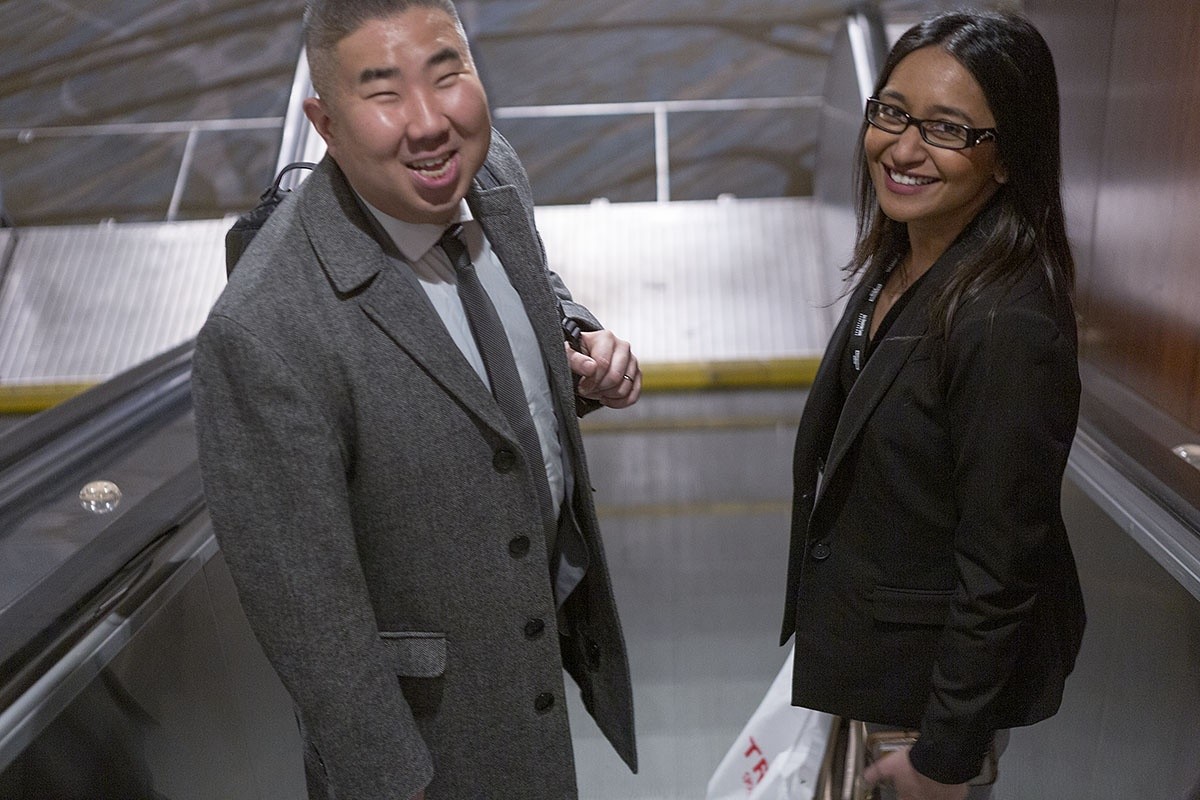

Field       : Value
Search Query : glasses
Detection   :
[865,95,999,150]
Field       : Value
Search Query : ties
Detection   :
[434,224,556,562]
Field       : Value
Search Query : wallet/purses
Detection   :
[862,732,996,790]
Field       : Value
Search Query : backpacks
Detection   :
[227,162,592,375]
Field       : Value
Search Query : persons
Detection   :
[192,0,641,800]
[779,13,1089,800]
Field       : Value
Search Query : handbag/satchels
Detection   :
[706,644,832,800]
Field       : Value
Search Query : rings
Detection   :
[624,374,634,383]
